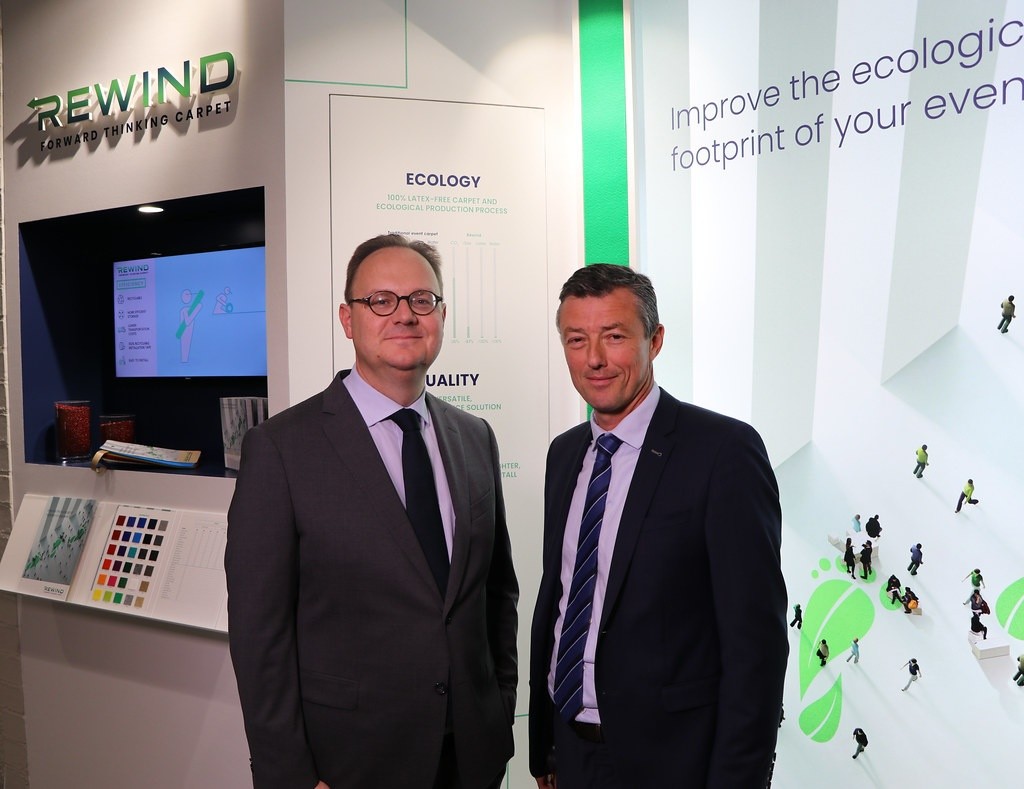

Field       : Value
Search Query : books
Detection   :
[220,397,268,471]
[100,439,201,468]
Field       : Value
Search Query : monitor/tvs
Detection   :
[110,240,268,383]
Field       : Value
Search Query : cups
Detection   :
[52,400,92,464]
[99,414,136,447]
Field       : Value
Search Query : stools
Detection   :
[887,590,894,599]
[912,608,923,616]
[970,629,983,645]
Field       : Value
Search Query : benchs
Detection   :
[972,641,1011,660]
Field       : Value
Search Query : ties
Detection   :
[380,408,450,604]
[553,432,625,718]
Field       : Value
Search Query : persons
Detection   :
[225,235,520,789]
[844,513,882,579]
[1012,653,1024,686]
[899,658,923,691]
[852,728,868,759]
[846,637,860,663]
[526,263,787,789]
[887,574,919,613]
[816,639,829,666]
[908,543,923,575]
[790,604,802,629]
[913,444,930,478]
[962,568,988,641]
[955,479,979,513]
[997,295,1016,333]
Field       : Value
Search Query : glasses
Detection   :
[348,288,444,317]
